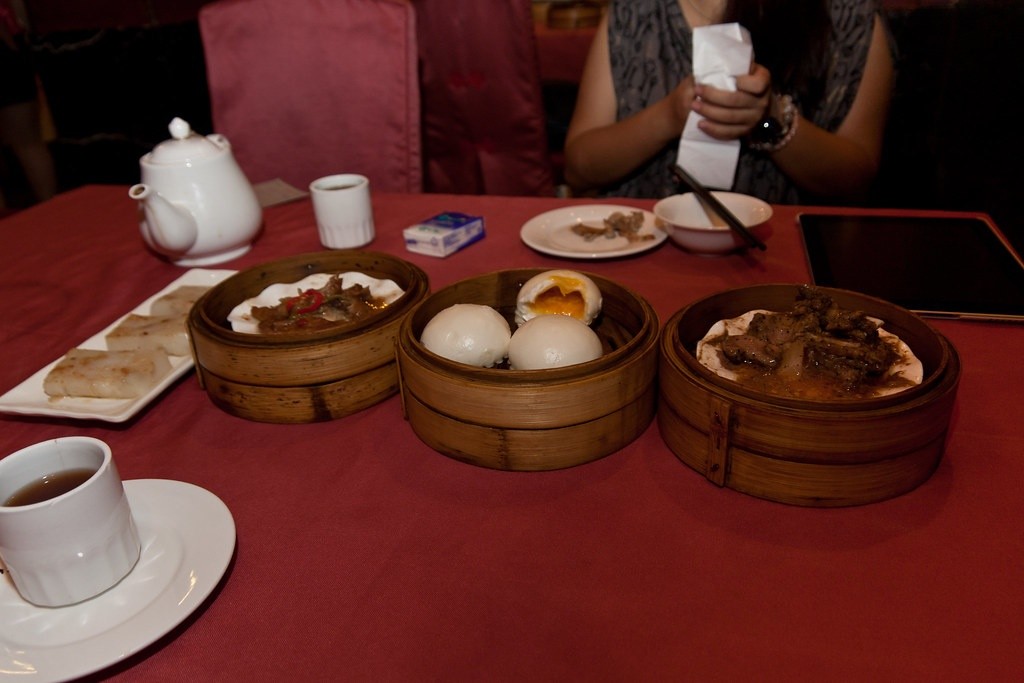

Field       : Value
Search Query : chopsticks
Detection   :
[668,163,767,252]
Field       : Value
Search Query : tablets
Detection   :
[795,213,1024,322]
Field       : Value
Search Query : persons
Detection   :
[559,0,895,200]
[0,0,59,216]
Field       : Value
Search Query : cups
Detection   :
[0,435,141,608]
[309,173,376,249]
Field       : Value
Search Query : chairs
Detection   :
[414,0,578,198]
[199,0,423,194]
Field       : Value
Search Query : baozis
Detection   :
[512,269,603,326]
[419,303,510,367]
[507,314,602,371]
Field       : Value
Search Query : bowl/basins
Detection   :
[653,191,773,258]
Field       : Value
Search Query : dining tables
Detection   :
[0,185,1024,683]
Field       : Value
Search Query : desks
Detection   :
[534,26,596,83]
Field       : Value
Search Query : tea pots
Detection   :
[128,117,263,267]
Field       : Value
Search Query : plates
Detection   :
[0,268,240,423]
[519,204,668,259]
[0,478,237,683]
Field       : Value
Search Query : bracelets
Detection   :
[743,93,798,154]
